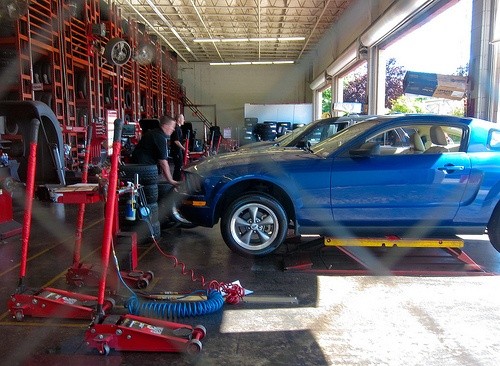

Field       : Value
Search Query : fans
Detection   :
[106,38,131,66]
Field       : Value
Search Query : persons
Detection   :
[170,113,186,181]
[129,116,182,241]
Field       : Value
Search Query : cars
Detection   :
[176,114,500,254]
